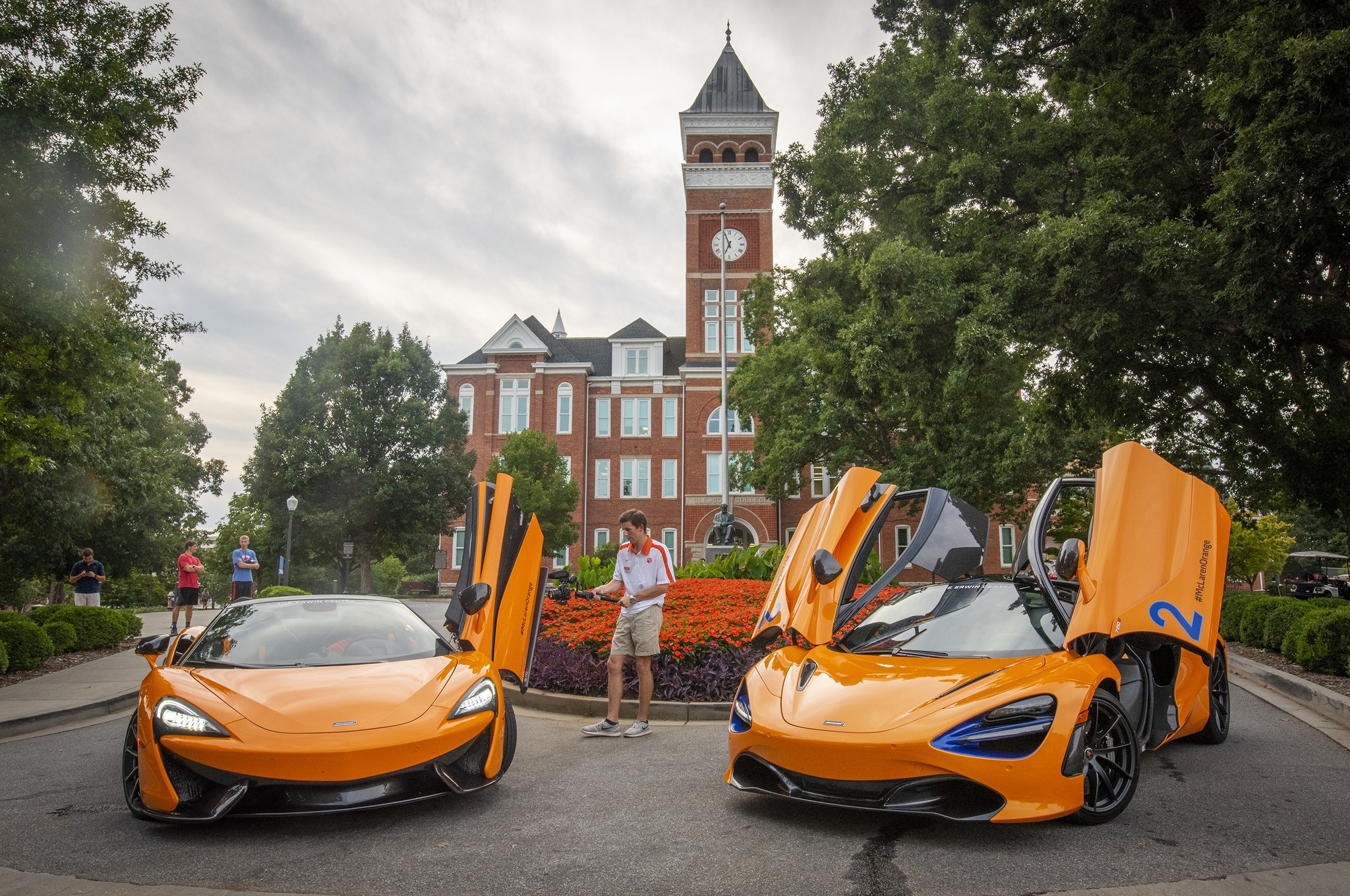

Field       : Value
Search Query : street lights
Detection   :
[282,494,302,589]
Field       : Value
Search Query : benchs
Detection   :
[1282,574,1313,594]
[401,581,431,596]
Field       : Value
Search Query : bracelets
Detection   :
[93,574,97,579]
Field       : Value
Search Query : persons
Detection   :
[580,508,677,738]
[1283,573,1303,584]
[70,548,105,607]
[201,588,210,610]
[1272,577,1277,586]
[1307,572,1328,585]
[230,535,260,601]
[712,503,735,544]
[170,540,204,635]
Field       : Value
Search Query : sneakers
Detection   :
[581,718,621,737]
[623,719,651,738]
[171,626,177,634]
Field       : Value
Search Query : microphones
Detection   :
[548,570,570,578]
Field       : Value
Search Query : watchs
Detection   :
[193,564,195,568]
[629,594,636,604]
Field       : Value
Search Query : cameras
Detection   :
[548,582,572,605]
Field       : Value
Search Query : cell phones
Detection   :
[86,571,89,573]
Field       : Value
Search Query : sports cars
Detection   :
[119,473,552,827]
[720,441,1230,825]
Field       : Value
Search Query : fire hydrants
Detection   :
[166,592,176,611]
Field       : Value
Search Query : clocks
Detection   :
[710,226,748,263]
